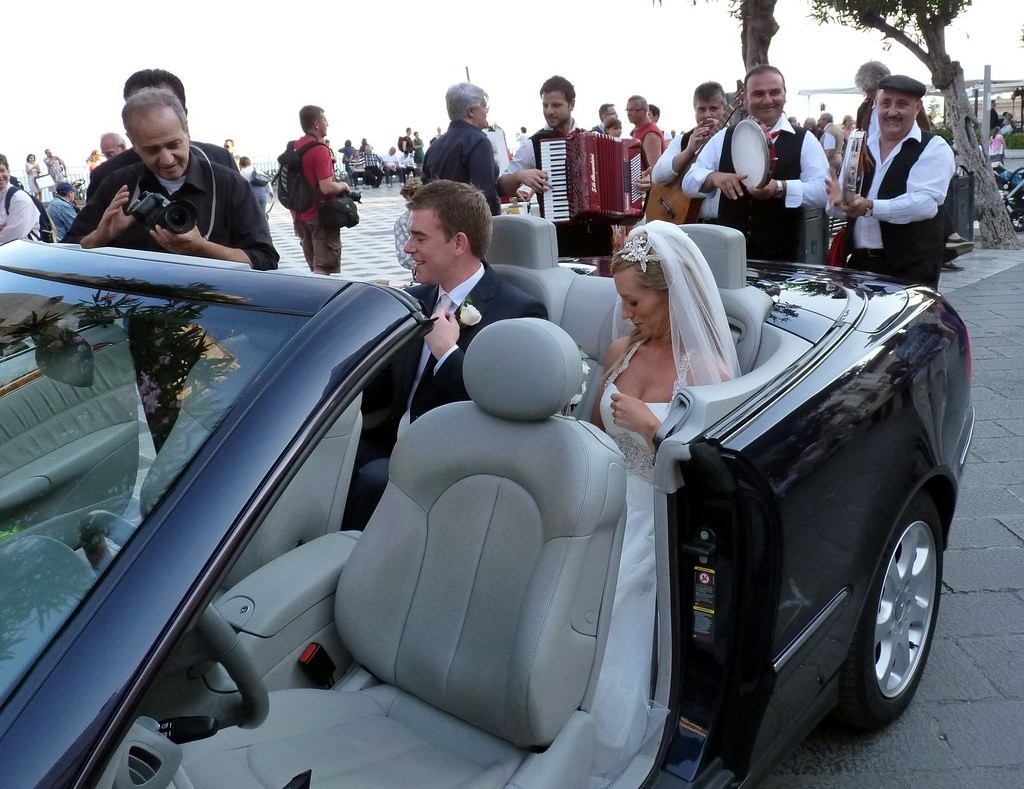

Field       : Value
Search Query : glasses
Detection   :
[224,145,229,148]
[607,112,616,115]
[625,108,644,112]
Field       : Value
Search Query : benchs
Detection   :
[347,163,411,189]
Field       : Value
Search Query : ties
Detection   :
[437,296,452,316]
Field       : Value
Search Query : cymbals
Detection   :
[841,128,868,202]
[730,115,778,190]
[851,139,860,152]
[846,171,856,185]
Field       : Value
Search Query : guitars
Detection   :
[644,84,745,225]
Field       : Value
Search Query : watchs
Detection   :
[774,181,785,200]
[863,199,875,218]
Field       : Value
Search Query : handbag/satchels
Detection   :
[279,141,332,213]
[316,198,360,229]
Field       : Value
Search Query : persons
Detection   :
[786,114,855,178]
[418,82,501,216]
[0,133,80,245]
[278,105,349,275]
[498,75,651,257]
[826,75,956,290]
[591,219,742,764]
[940,208,974,271]
[855,62,930,143]
[56,69,280,454]
[987,100,1013,165]
[589,96,675,176]
[340,178,551,532]
[326,127,443,188]
[681,65,832,264]
[394,176,423,282]
[652,82,730,224]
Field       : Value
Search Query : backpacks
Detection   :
[5,185,57,243]
[398,138,405,152]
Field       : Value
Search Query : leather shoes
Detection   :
[941,261,964,272]
[946,237,975,249]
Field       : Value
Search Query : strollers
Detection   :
[989,159,1024,233]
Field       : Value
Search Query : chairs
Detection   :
[573,222,773,421]
[489,214,576,325]
[75,332,362,599]
[162,317,626,788]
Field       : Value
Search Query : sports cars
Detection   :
[0,240,977,789]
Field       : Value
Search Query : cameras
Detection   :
[131,193,199,234]
[337,190,364,205]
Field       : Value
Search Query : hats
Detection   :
[57,182,78,196]
[879,73,926,97]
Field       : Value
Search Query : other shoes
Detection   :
[356,185,360,189]
[362,184,367,188]
[386,184,392,188]
[350,187,355,190]
[373,184,379,188]
[400,183,404,187]
